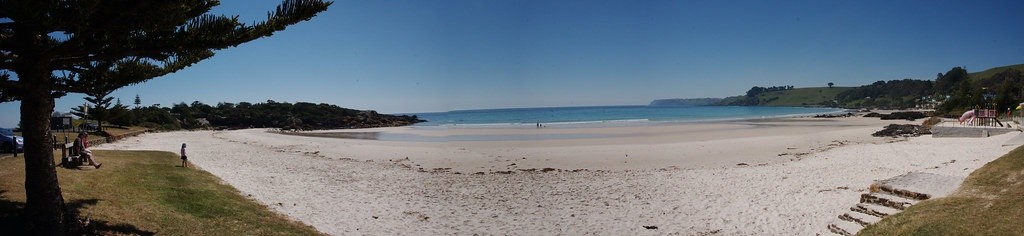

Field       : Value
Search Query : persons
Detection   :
[181,143,189,167]
[73,132,102,169]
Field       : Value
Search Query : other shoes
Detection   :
[89,163,94,165]
[94,163,102,169]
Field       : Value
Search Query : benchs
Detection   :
[62,141,87,167]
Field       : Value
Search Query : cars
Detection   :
[0,126,25,154]
